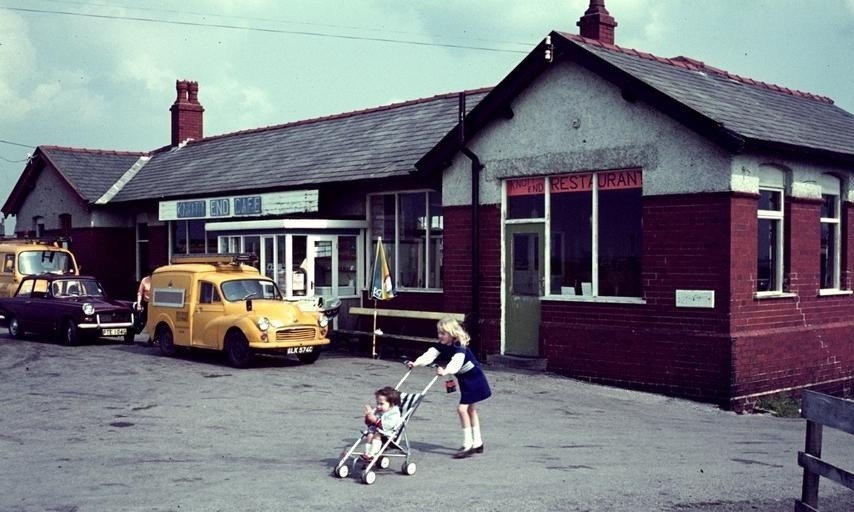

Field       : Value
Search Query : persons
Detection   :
[128,262,159,343]
[404,317,491,458]
[360,387,403,460]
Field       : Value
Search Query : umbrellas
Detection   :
[368,235,397,358]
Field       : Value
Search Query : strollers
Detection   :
[333,360,440,485]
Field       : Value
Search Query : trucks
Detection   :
[147,252,330,367]
[0,241,79,297]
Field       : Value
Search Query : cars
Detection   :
[0,273,138,344]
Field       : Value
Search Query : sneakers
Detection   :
[455,443,484,458]
[359,452,373,461]
